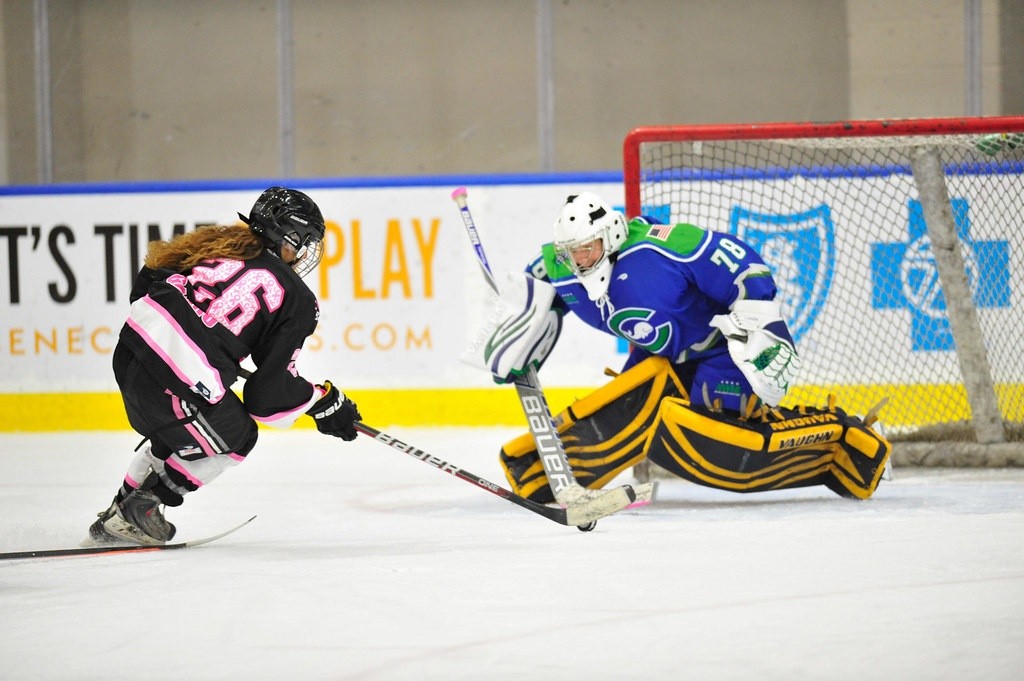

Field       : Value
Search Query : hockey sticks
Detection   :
[451,187,657,532]
[1,514,260,560]
[235,367,636,526]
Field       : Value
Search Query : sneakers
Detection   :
[90,471,183,544]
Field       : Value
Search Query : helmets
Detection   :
[555,192,628,302]
[238,186,326,278]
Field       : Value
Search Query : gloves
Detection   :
[306,380,362,442]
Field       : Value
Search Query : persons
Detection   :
[487,194,892,481]
[89,187,363,544]
